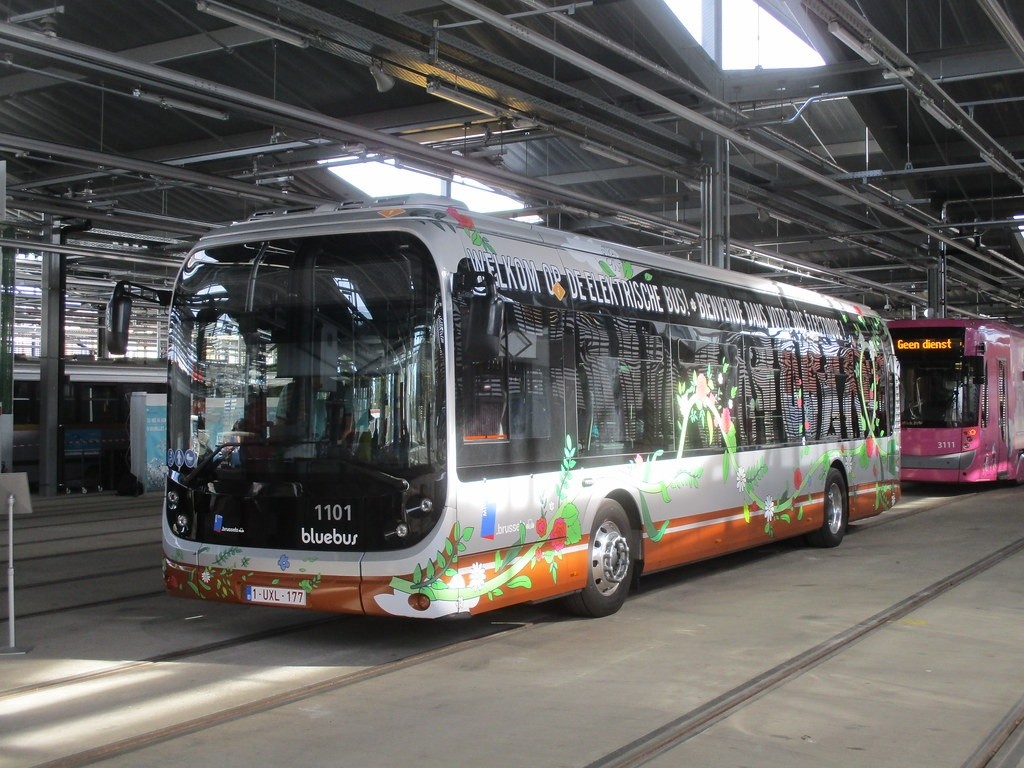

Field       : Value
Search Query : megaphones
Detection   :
[368,65,394,93]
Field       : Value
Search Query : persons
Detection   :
[97,384,132,491]
[271,353,355,444]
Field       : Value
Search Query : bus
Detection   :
[12,354,373,495]
[883,317,1024,485]
[105,192,904,622]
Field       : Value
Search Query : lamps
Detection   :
[196,0,310,49]
[870,251,887,259]
[827,22,879,65]
[426,81,501,117]
[910,265,924,272]
[920,100,954,129]
[882,67,914,80]
[990,297,1000,301]
[979,152,1005,173]
[769,213,791,224]
[133,88,228,121]
[512,120,537,128]
[579,143,632,166]
